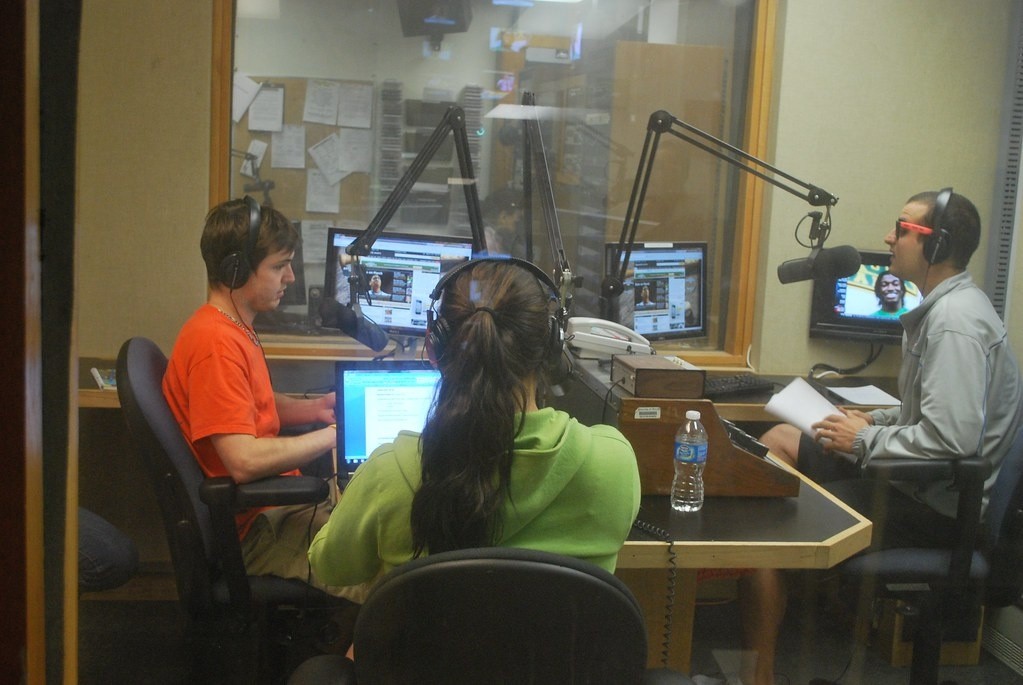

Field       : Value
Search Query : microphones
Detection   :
[777,245,861,285]
[318,297,390,352]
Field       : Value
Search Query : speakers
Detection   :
[397,0,473,37]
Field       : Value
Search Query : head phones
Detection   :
[219,196,261,289]
[923,187,954,265]
[425,254,572,368]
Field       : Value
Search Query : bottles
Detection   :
[671,410,708,513]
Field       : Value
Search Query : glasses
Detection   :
[895,219,933,240]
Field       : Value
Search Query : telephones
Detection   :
[564,317,655,360]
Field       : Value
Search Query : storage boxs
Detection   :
[402,132,452,159]
[402,191,451,225]
[405,99,456,127]
[405,165,455,185]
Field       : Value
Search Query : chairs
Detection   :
[115,336,1023,685]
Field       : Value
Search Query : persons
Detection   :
[698,188,1023,685]
[160,197,378,661]
[307,257,640,595]
[366,274,391,298]
[634,285,657,306]
[865,271,910,320]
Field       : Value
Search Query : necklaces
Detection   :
[207,303,259,346]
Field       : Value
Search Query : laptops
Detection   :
[335,358,445,494]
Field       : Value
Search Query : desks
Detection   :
[326,416,872,685]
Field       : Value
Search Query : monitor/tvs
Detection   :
[277,220,307,306]
[320,227,477,338]
[605,242,708,341]
[809,250,923,345]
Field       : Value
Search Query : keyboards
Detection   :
[705,375,776,400]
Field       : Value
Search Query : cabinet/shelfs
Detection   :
[491,30,735,323]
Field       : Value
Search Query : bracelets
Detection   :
[330,423,336,428]
